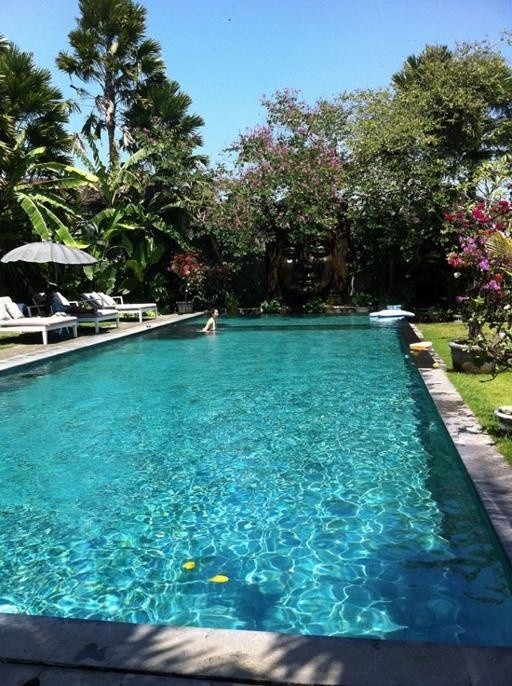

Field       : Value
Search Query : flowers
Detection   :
[168,254,223,303]
[441,155,512,367]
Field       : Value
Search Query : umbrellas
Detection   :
[0,240,98,295]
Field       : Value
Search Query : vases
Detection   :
[176,302,193,313]
[449,340,497,374]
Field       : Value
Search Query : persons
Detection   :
[45,285,99,314]
[201,307,220,331]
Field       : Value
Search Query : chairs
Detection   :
[0,292,158,345]
